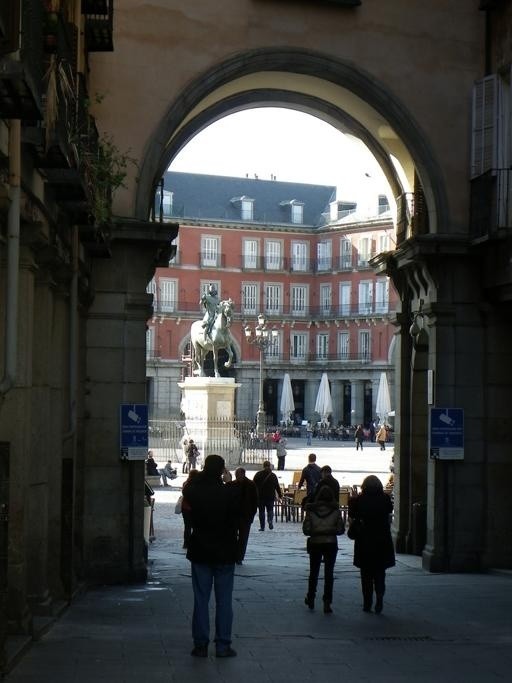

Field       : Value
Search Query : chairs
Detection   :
[271,471,392,526]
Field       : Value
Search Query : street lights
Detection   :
[244,312,278,439]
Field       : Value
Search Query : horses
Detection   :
[177,296,234,378]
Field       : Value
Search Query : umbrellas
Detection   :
[376,372,391,425]
[315,373,333,425]
[280,373,295,422]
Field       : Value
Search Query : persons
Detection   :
[182,455,283,658]
[199,284,220,345]
[298,454,396,614]
[146,439,200,487]
[235,421,391,471]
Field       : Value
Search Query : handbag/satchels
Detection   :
[346,520,363,541]
[306,537,311,555]
[174,495,185,514]
[257,494,260,507]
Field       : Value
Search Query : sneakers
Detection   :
[216,648,237,658]
[190,646,208,658]
[322,606,333,614]
[362,608,372,613]
[374,593,383,614]
[304,597,316,609]
[258,529,264,531]
[269,523,273,530]
